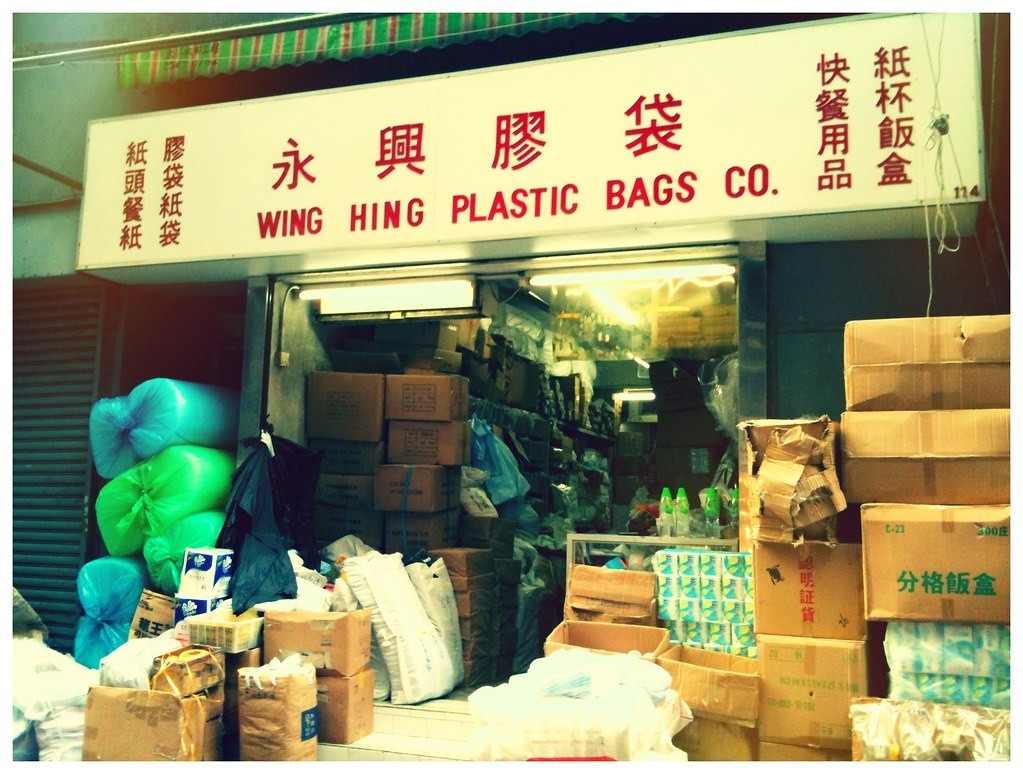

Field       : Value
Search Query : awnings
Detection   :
[117,13,659,93]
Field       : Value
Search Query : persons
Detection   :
[600,503,660,570]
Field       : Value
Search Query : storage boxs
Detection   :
[81,313,1010,762]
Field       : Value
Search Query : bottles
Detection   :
[675,489,689,539]
[705,488,721,539]
[728,490,739,538]
[660,487,673,537]
